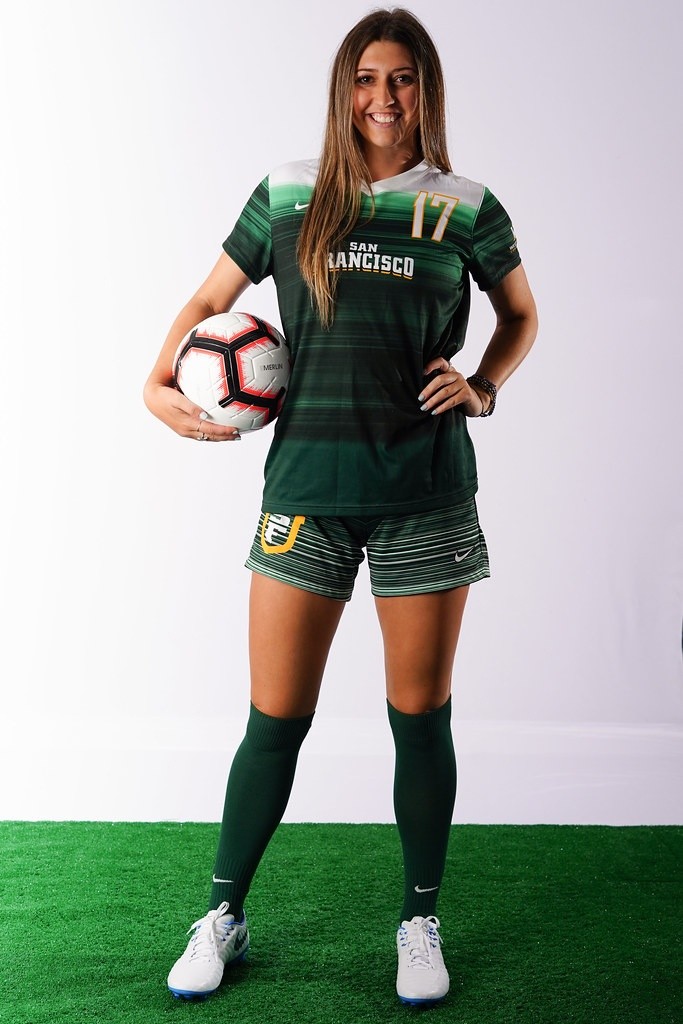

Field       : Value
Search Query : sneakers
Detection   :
[168,909,250,1000]
[395,916,449,1006]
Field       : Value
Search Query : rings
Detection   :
[197,420,203,432]
[197,433,208,441]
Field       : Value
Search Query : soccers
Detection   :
[174,313,290,435]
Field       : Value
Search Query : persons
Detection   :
[140,5,536,1006]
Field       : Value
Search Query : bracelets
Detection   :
[465,375,497,418]
